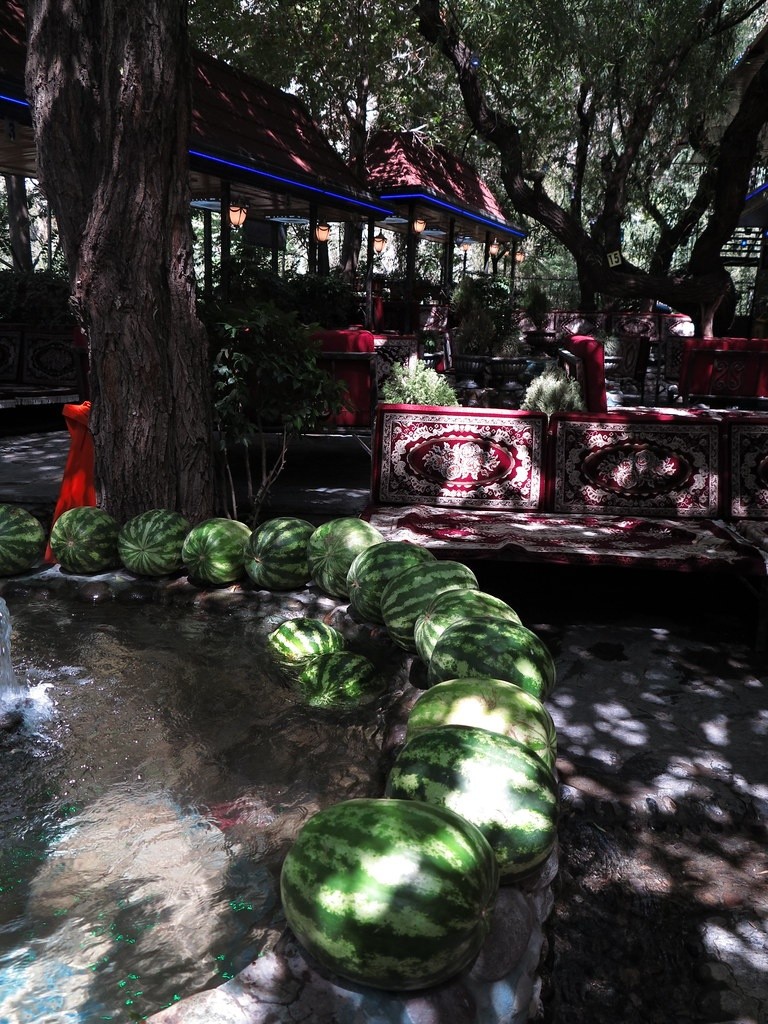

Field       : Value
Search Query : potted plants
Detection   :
[524,283,556,347]
[450,321,494,390]
[491,338,528,390]
[603,335,624,385]
[422,332,445,370]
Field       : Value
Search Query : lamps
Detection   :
[315,218,332,245]
[375,228,387,255]
[230,198,249,230]
[414,216,426,236]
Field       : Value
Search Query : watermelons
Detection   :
[50,506,557,768]
[0,505,43,573]
[384,725,559,883]
[280,798,500,991]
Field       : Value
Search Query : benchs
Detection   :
[212,297,768,675]
[0,323,80,410]
